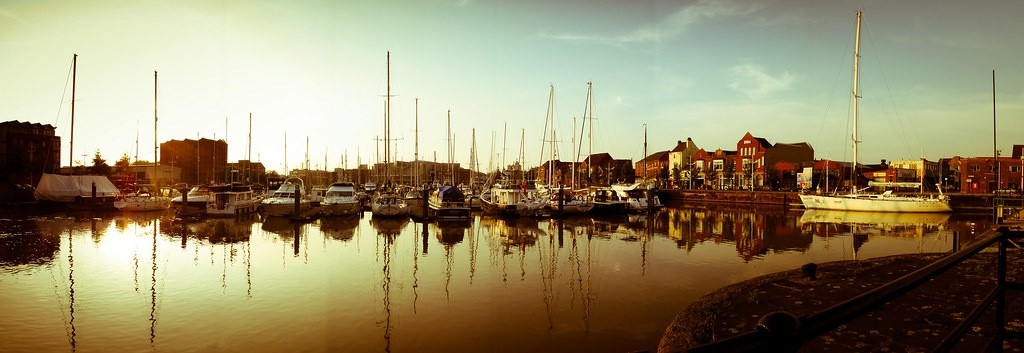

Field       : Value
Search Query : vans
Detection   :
[718,184,729,190]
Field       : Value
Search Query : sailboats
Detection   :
[799,208,951,261]
[29,51,664,223]
[797,10,954,215]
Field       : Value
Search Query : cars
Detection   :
[738,184,754,190]
[993,188,1016,194]
[779,184,796,192]
[673,185,682,189]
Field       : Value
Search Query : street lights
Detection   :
[945,177,948,193]
[748,161,758,191]
[686,162,695,190]
